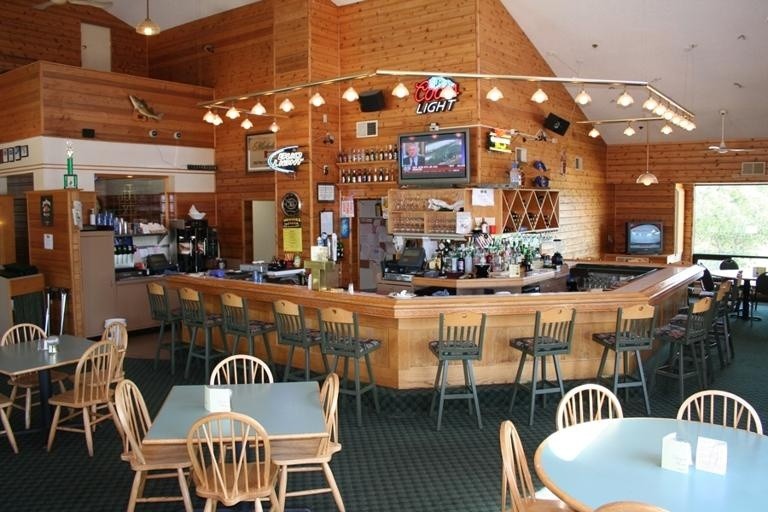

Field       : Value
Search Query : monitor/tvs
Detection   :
[625,220,664,255]
[397,127,471,188]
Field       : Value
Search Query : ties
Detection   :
[412,156,416,168]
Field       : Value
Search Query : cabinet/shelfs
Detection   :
[335,158,398,187]
[495,189,559,236]
[387,187,501,238]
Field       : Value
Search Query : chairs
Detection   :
[135,1,161,35]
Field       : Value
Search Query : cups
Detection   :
[348,283,354,295]
[253,270,263,282]
[490,225,496,234]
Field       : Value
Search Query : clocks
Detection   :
[281,192,302,217]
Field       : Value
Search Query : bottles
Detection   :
[504,195,550,233]
[90,209,128,235]
[509,163,522,186]
[480,217,488,233]
[341,167,394,183]
[114,246,133,265]
[338,143,398,163]
[47,336,59,355]
[428,237,553,274]
[317,232,344,258]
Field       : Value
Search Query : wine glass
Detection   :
[583,276,610,289]
[392,198,456,233]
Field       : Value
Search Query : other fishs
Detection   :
[129,95,164,122]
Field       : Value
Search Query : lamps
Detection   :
[636,143,659,186]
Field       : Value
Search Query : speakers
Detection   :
[358,90,385,112]
[544,112,571,137]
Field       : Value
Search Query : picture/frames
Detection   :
[0,144,28,165]
[320,210,334,237]
[40,195,53,227]
[316,182,336,203]
[245,130,276,172]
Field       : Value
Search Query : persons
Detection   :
[42,200,50,216]
[403,143,425,166]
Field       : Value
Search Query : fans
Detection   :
[35,0,112,10]
[703,117,755,153]
